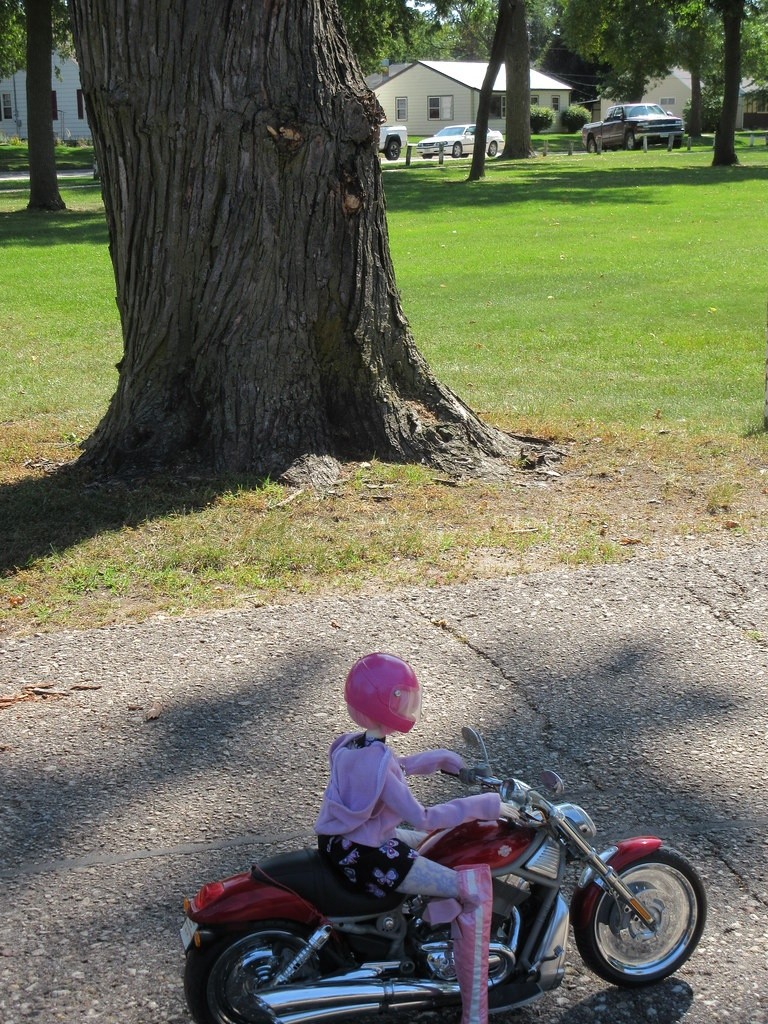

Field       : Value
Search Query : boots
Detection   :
[422,862,493,1024]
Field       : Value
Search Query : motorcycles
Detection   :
[176,725,708,1024]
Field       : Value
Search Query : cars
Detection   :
[415,123,506,161]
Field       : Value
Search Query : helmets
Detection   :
[344,653,422,732]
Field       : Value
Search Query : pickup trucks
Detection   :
[580,102,685,155]
[378,124,409,162]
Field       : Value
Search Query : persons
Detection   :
[313,653,521,1023]
